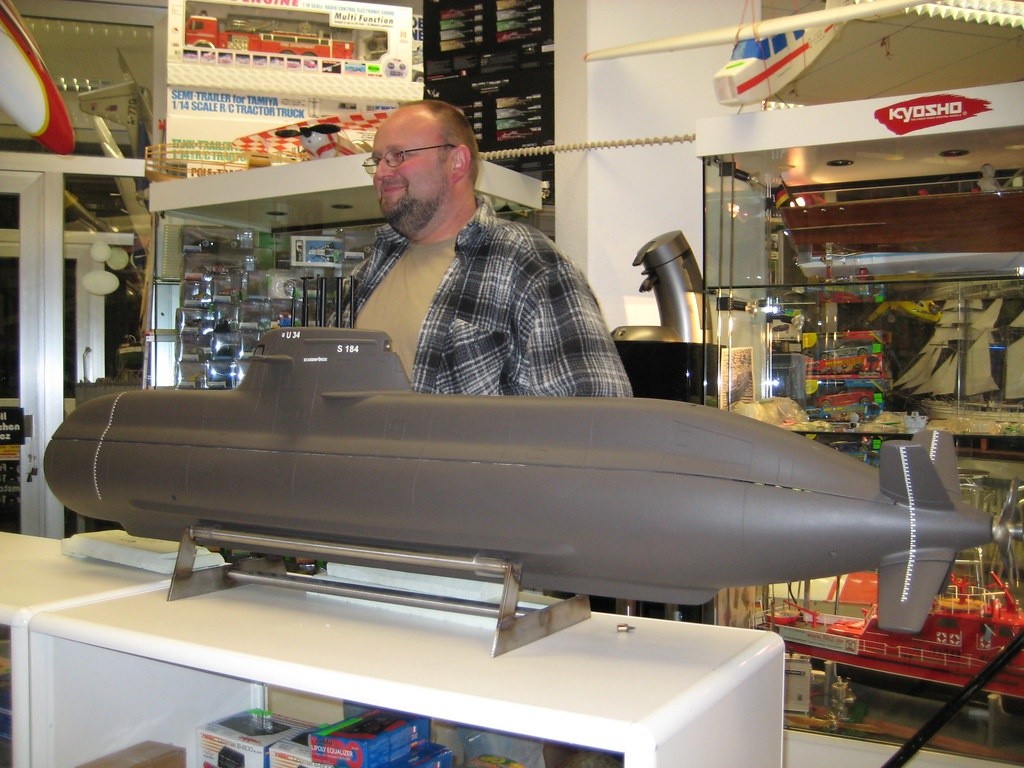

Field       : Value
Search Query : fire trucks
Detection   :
[185,15,355,66]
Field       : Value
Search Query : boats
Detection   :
[747,570,1024,699]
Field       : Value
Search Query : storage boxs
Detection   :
[809,329,892,409]
[785,660,810,712]
[165,0,556,203]
[86,706,547,768]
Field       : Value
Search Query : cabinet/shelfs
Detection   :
[148,151,542,387]
[31,564,786,768]
[0,531,230,768]
[694,82,1024,768]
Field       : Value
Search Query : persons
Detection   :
[324,100,634,768]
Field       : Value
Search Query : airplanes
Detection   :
[231,109,395,160]
[583,0,938,107]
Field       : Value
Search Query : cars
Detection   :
[819,340,873,361]
[814,388,875,408]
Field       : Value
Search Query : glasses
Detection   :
[362,143,455,174]
